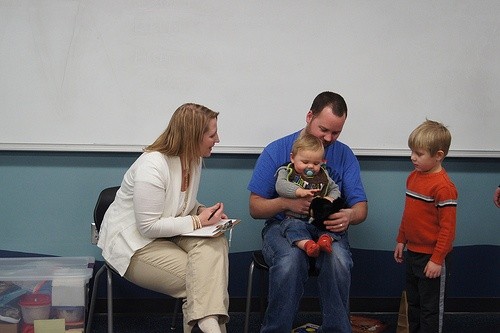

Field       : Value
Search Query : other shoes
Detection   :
[304,241,318,258]
[191,321,204,333]
[317,234,333,253]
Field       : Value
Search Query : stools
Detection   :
[244,250,351,333]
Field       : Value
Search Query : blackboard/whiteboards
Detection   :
[0,0,500,158]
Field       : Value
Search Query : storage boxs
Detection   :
[0,256,95,333]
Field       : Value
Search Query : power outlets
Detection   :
[91,223,99,245]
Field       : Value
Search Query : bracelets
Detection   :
[192,215,202,229]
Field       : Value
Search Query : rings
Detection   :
[340,223,342,226]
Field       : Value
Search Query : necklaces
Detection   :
[182,177,187,211]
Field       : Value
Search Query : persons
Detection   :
[274,134,344,256]
[97,103,230,333]
[247,91,369,333]
[394,120,457,333]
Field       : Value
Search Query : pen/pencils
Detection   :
[208,206,220,220]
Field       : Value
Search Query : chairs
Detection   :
[85,185,181,333]
